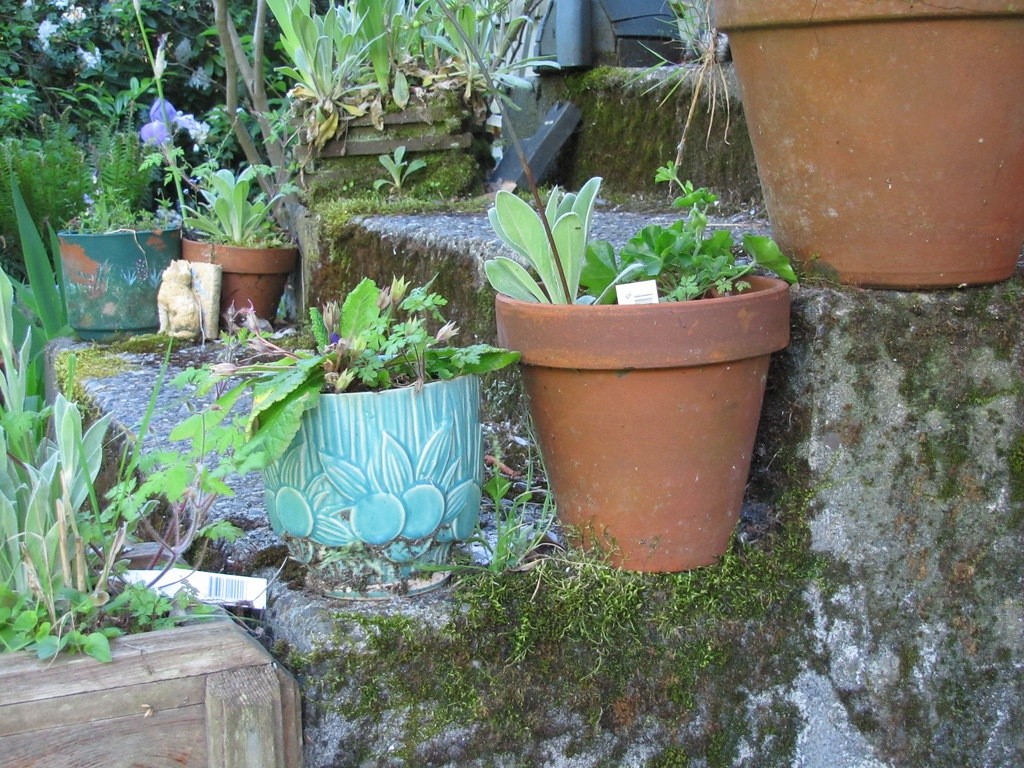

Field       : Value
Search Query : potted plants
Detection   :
[268,0,562,199]
[482,176,798,573]
[199,276,521,601]
[58,131,181,343]
[0,268,304,768]
[181,165,299,333]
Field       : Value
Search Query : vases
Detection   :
[717,0,1024,287]
